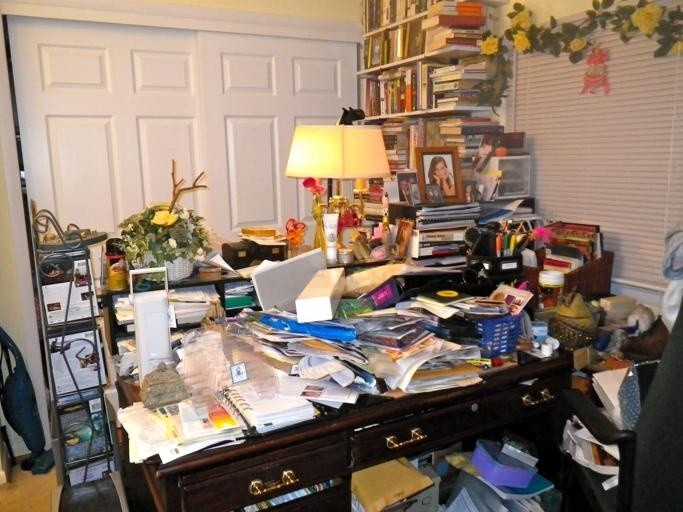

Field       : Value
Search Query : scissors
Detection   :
[285,218,308,245]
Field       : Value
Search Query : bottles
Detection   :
[103,251,127,292]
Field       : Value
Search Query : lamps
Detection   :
[284,124,390,213]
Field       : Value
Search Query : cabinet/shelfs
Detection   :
[355,0,509,122]
[31,209,114,490]
[103,256,391,357]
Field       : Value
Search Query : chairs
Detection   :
[557,296,683,512]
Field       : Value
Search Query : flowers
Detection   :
[471,1,682,119]
[118,202,213,284]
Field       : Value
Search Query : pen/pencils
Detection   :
[495,233,530,258]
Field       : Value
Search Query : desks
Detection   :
[113,346,571,509]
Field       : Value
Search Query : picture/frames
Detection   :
[415,146,466,205]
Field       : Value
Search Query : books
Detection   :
[474,472,555,501]
[349,1,607,299]
[109,279,359,466]
[487,283,534,316]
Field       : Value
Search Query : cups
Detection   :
[537,270,565,310]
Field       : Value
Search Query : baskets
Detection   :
[547,314,598,351]
[475,313,523,358]
